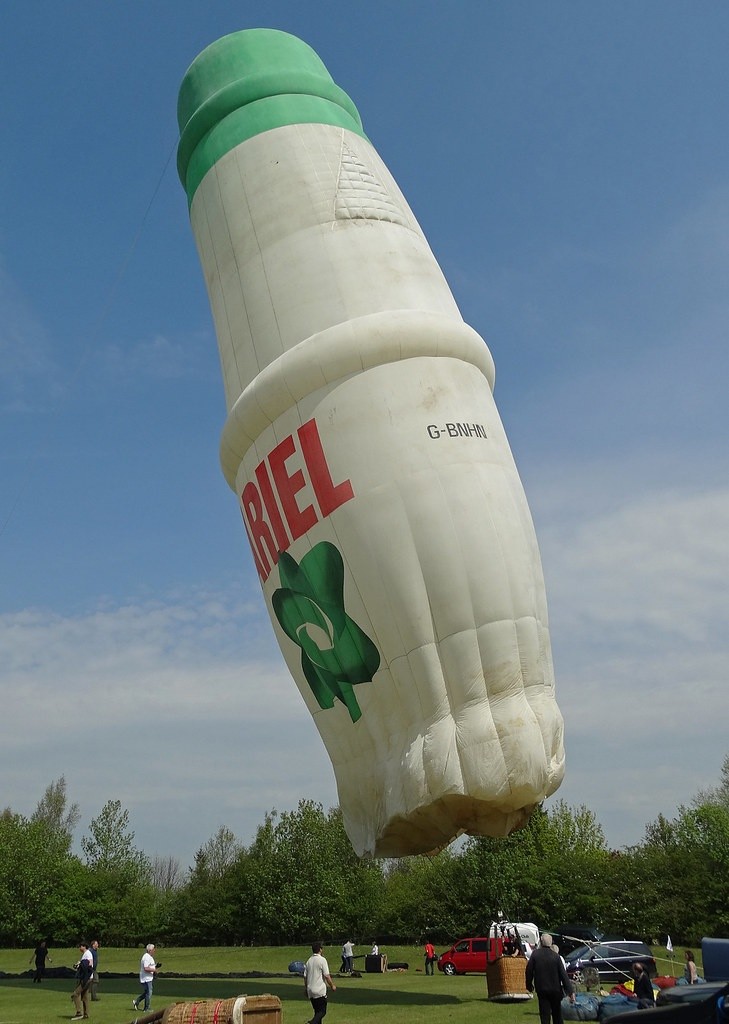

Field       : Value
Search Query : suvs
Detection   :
[563,940,659,985]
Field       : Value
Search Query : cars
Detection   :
[511,941,534,961]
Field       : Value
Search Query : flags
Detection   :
[666,935,673,952]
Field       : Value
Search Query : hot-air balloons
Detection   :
[175,28,569,951]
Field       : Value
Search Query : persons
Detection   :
[303,943,336,1024]
[424,940,436,975]
[88,940,101,1001]
[525,934,576,1024]
[632,962,654,1009]
[339,940,355,973]
[501,934,525,957]
[29,940,52,983]
[685,950,698,985]
[371,941,378,955]
[71,942,93,1021]
[133,944,158,1012]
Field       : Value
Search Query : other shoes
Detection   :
[33,980,36,983]
[92,998,100,1001]
[38,981,41,983]
[133,999,139,1011]
[143,1008,153,1012]
[71,1015,83,1020]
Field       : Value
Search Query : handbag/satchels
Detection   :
[432,954,438,960]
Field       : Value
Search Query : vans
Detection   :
[488,921,541,952]
[438,937,515,976]
